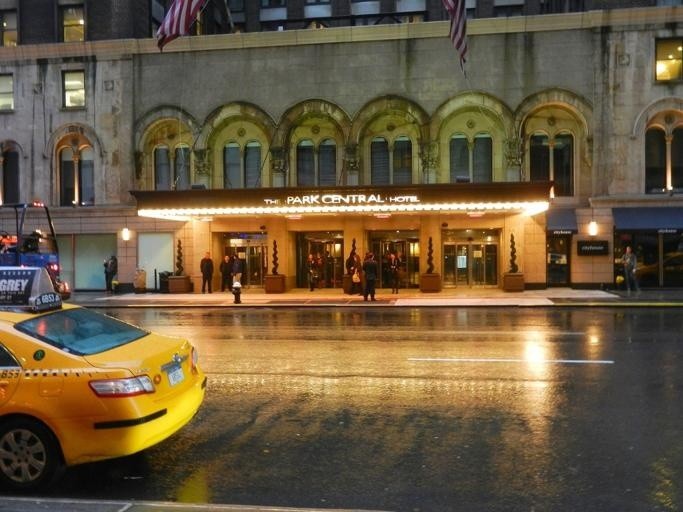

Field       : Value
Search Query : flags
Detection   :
[155,0,209,53]
[442,1,469,81]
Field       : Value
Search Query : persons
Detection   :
[199,251,213,294]
[232,255,242,292]
[305,254,315,291]
[218,254,232,292]
[388,253,399,294]
[620,246,642,293]
[315,252,324,289]
[102,254,116,292]
[347,254,363,296]
[362,253,376,302]
[323,251,333,288]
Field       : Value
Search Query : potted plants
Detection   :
[167,239,192,294]
[342,237,363,295]
[262,238,288,293]
[418,235,444,292]
[501,232,527,293]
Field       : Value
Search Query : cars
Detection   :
[2,265,214,497]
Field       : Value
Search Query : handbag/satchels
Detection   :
[352,271,361,283]
